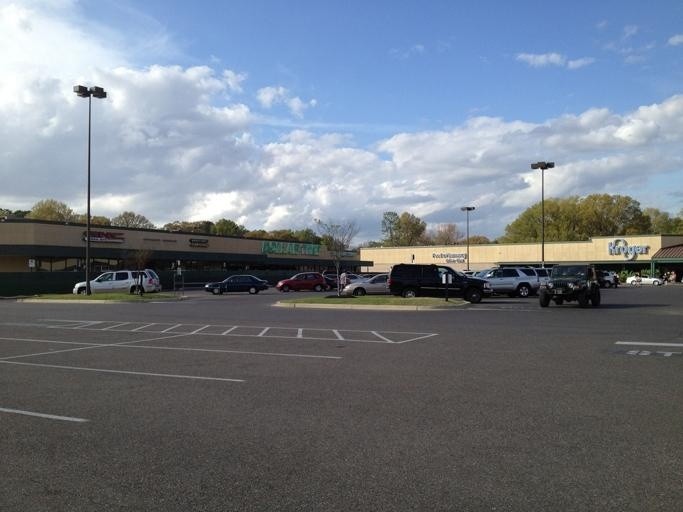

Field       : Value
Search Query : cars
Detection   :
[323,269,354,290]
[276,271,326,291]
[472,264,552,297]
[203,274,269,294]
[597,269,619,288]
[341,272,389,296]
[626,273,663,285]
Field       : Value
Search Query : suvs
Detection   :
[539,262,601,307]
[73,267,161,295]
[389,263,493,302]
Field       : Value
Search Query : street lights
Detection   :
[75,85,107,296]
[531,162,554,268]
[461,206,476,272]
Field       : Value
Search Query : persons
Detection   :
[634,274,641,287]
[668,270,677,283]
[322,269,328,276]
[339,269,347,292]
[613,271,619,288]
